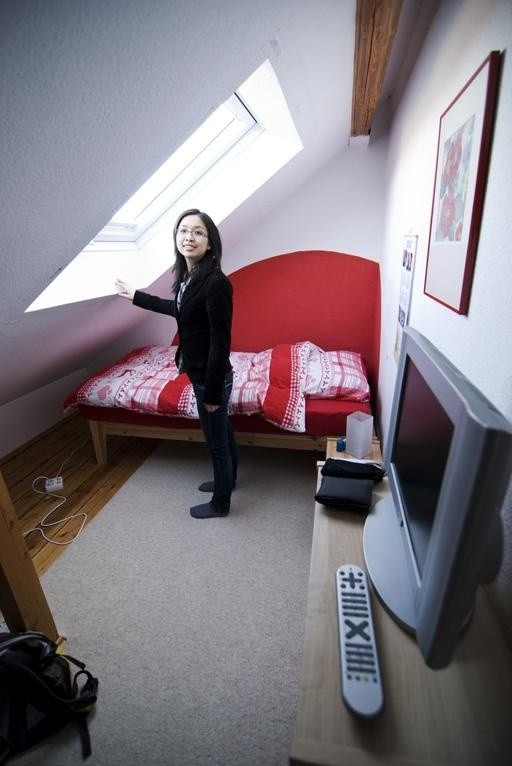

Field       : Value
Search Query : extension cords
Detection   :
[45,477,64,493]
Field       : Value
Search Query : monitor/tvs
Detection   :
[362,325,511,670]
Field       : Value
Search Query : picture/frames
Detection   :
[423,50,501,316]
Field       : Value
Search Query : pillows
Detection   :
[304,350,371,403]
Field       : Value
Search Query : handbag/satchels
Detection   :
[314,476,374,513]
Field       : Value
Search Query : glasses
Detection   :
[177,228,208,238]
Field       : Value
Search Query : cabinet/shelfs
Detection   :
[294,461,512,766]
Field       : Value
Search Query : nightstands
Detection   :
[326,437,383,462]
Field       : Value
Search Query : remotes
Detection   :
[335,562,385,723]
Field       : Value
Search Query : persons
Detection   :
[113,208,240,520]
